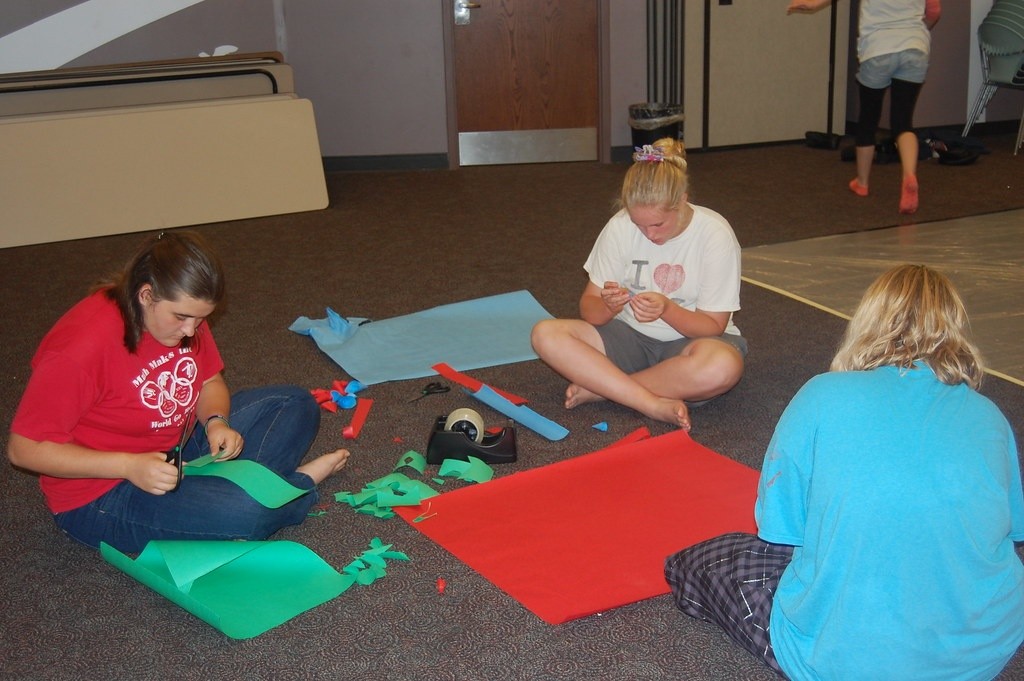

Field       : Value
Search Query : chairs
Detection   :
[961,0,1024,154]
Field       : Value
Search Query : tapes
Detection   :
[444,408,485,444]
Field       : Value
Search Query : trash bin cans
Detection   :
[629,103,683,152]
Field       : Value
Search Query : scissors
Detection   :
[160,412,199,493]
[407,382,450,404]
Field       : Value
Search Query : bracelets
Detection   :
[204,415,230,434]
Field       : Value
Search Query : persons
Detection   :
[787,0,941,214]
[665,263,1024,681]
[530,138,748,432]
[6,232,349,553]
